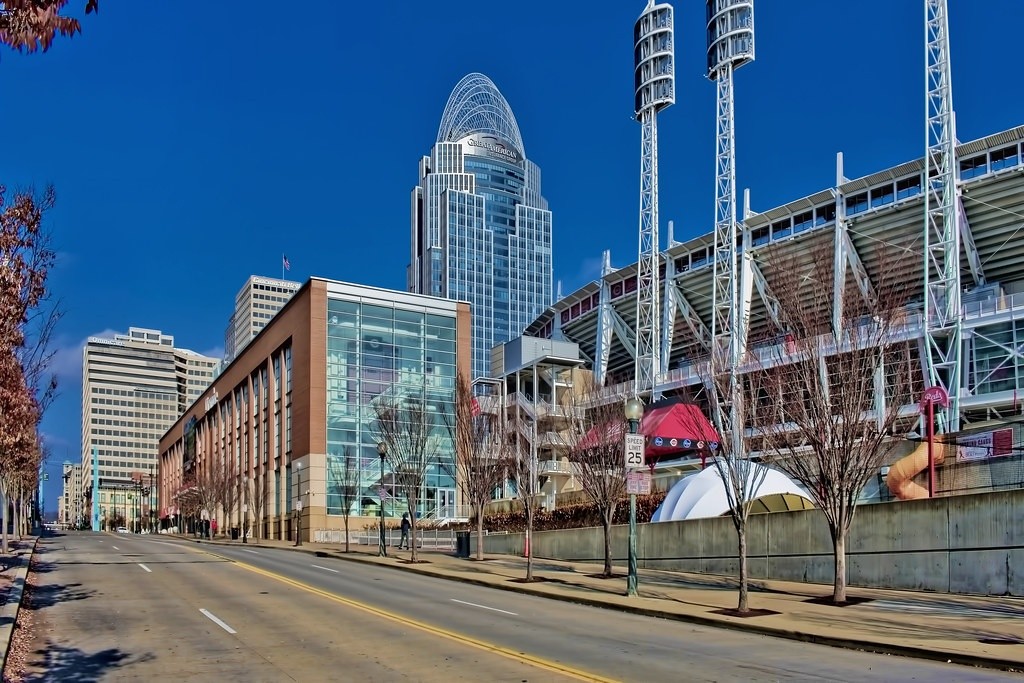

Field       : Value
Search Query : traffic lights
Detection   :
[141,489,144,496]
[145,487,148,495]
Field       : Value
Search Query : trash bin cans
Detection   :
[231,528,239,540]
[456,531,471,558]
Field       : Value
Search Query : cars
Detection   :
[40,527,53,537]
[117,527,131,533]
[74,523,92,531]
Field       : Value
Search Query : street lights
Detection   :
[113,486,116,531]
[625,400,643,597]
[149,466,153,534]
[924,398,940,497]
[376,442,387,557]
[242,476,247,543]
[296,462,303,546]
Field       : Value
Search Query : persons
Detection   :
[195,518,217,539]
[399,514,411,550]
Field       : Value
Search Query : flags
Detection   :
[284,255,290,271]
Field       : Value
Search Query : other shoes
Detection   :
[407,547,409,550]
[399,547,402,549]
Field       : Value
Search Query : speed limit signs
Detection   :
[625,433,645,468]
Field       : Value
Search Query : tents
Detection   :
[575,403,723,488]
[650,460,816,523]
